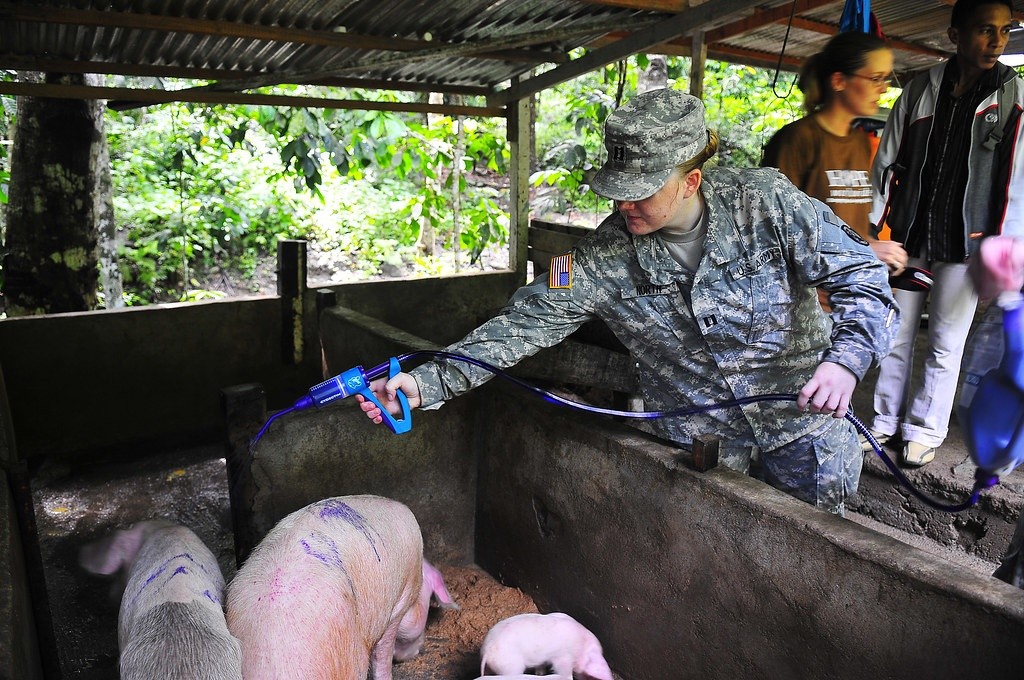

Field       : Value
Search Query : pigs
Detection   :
[480,612,615,680]
[80,516,244,680]
[226,494,459,680]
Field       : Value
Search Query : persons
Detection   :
[761,33,909,276]
[858,0,1023,464]
[354,89,901,512]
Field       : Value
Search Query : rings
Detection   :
[894,262,900,268]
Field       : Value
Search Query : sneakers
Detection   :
[857,428,893,452]
[903,440,936,465]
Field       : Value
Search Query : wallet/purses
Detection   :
[888,266,935,291]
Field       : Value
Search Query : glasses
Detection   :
[839,72,893,87]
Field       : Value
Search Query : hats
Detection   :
[589,88,706,202]
